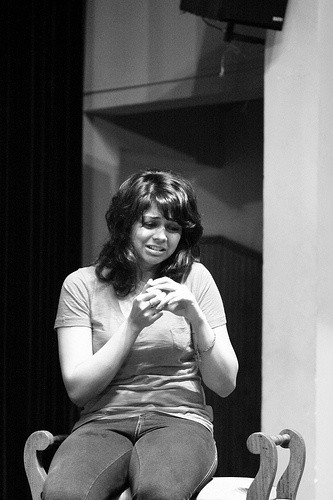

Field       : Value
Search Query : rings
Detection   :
[149,301,153,308]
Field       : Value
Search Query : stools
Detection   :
[23,429,306,500]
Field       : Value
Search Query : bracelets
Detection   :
[197,331,216,360]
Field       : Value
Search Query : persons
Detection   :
[42,168,238,500]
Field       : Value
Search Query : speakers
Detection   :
[179,0,288,30]
